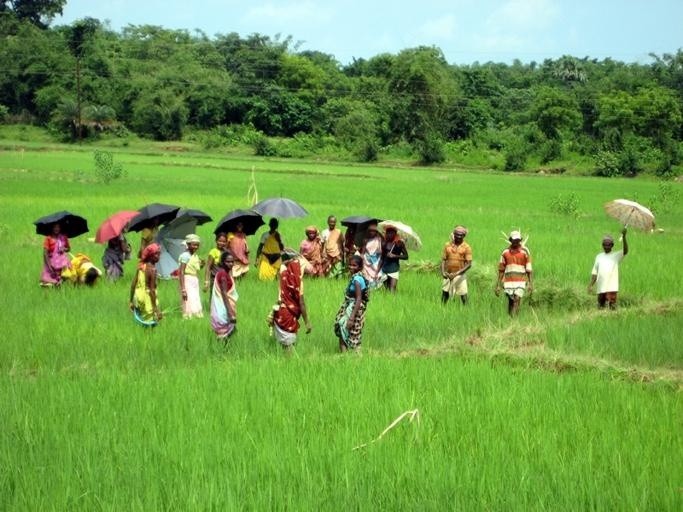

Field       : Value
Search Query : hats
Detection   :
[510,231,521,240]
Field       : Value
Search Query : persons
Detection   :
[210,252,238,351]
[361,225,382,291]
[102,236,132,283]
[588,228,629,310]
[442,226,472,305]
[254,218,284,282]
[179,234,206,320]
[334,256,368,353]
[39,226,71,287]
[300,226,321,279]
[228,222,249,278]
[130,243,160,332]
[138,226,162,259]
[208,233,227,314]
[344,225,364,269]
[320,216,345,279]
[267,260,301,348]
[494,231,532,317]
[374,225,408,292]
[61,253,101,286]
[278,248,314,332]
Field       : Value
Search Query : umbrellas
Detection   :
[341,217,383,225]
[604,199,655,241]
[95,210,141,242]
[120,203,181,232]
[174,209,212,226]
[33,210,89,238]
[376,220,422,254]
[213,208,263,237]
[249,197,309,232]
[145,213,204,280]
